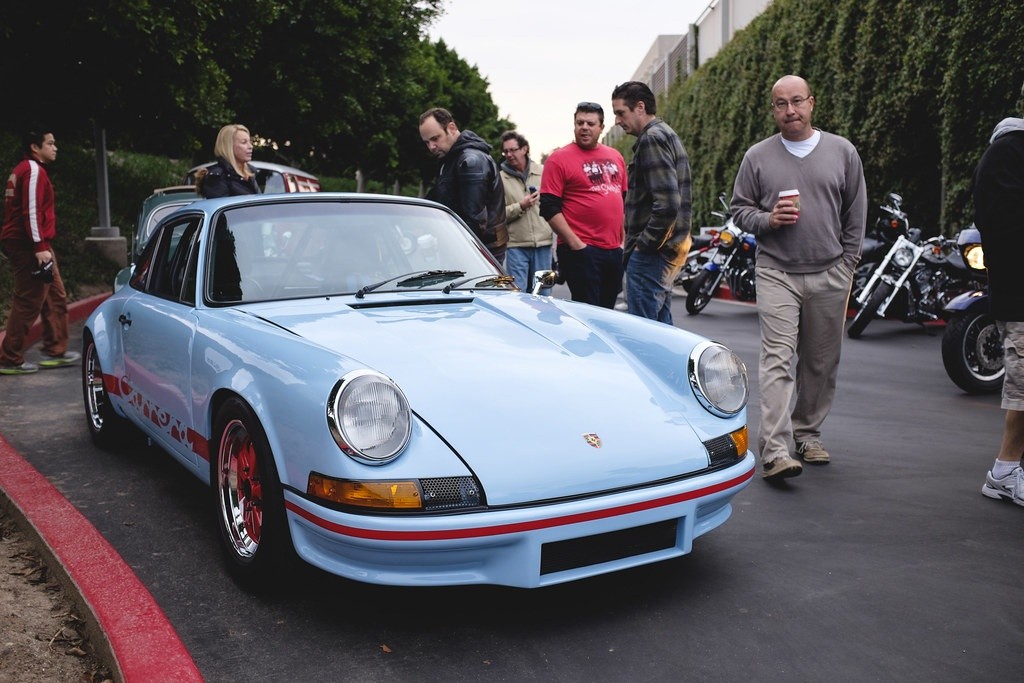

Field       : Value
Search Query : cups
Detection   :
[778,189,800,221]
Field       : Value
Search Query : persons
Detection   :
[730,74,869,479]
[419,107,509,264]
[499,130,553,297]
[0,127,81,374]
[972,117,1024,505]
[539,101,627,310]
[612,81,692,325]
[198,124,260,196]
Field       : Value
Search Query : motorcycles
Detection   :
[670,191,890,316]
[940,227,1006,395]
[846,192,971,340]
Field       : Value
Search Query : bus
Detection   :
[183,161,322,204]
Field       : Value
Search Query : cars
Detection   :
[125,185,210,266]
[80,190,756,589]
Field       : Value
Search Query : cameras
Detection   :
[31,260,54,282]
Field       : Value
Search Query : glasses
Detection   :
[773,94,811,109]
[503,147,521,153]
[579,103,605,122]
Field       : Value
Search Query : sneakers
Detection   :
[0,360,38,374]
[38,351,82,368]
[982,453,1024,506]
[762,456,803,481]
[795,441,830,463]
